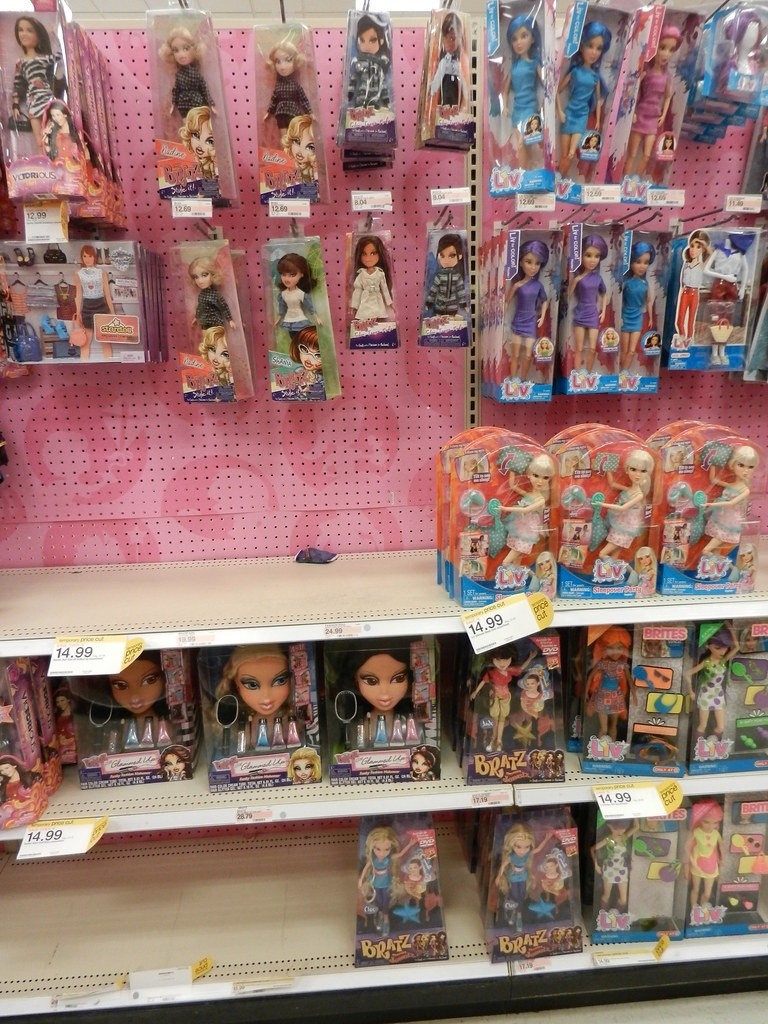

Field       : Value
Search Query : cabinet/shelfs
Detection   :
[0,0,768,1024]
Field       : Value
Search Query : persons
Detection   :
[717,11,762,89]
[585,627,638,742]
[619,241,656,368]
[470,644,539,754]
[158,28,220,126]
[206,643,306,747]
[0,757,32,805]
[346,14,393,110]
[701,236,749,367]
[699,444,760,558]
[348,233,396,322]
[425,235,470,322]
[556,20,613,177]
[428,12,468,109]
[330,639,420,740]
[262,42,319,139]
[73,648,176,743]
[357,800,726,939]
[686,619,740,744]
[569,234,609,373]
[504,239,549,381]
[272,253,324,339]
[42,104,84,163]
[187,256,237,336]
[623,24,682,178]
[590,450,655,560]
[500,15,545,169]
[494,454,556,566]
[52,688,75,739]
[8,17,66,147]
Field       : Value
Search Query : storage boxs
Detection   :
[0,0,768,969]
